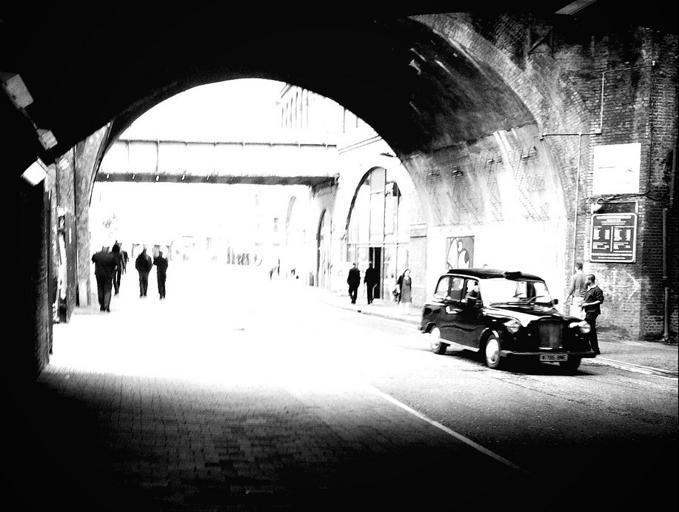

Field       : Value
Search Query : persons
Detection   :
[577,274,604,355]
[394,268,412,316]
[456,239,470,271]
[468,280,481,300]
[363,262,376,304]
[103,243,130,295]
[153,250,168,299]
[135,248,153,297]
[92,246,114,313]
[227,244,297,282]
[563,262,589,327]
[346,262,361,304]
[444,277,461,300]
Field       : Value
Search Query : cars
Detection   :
[417,268,596,371]
